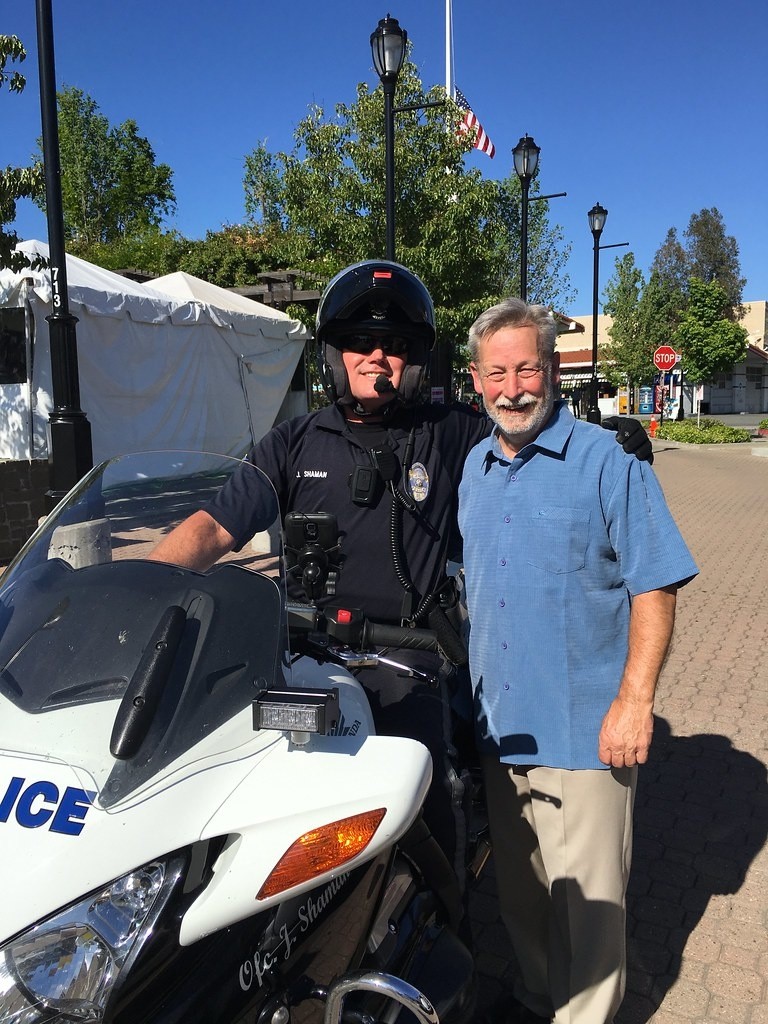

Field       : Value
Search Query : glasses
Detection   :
[334,332,411,356]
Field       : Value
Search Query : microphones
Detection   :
[374,376,411,406]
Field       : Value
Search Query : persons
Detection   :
[456,298,699,1024]
[149,257,654,1024]
[571,388,581,419]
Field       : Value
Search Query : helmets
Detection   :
[315,258,437,357]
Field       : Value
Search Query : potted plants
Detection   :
[758,418,768,438]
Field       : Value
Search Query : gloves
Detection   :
[586,406,654,465]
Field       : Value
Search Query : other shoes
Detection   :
[442,951,478,1024]
[474,994,553,1024]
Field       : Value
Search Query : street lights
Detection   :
[368,13,447,259]
[587,202,630,424]
[510,130,569,310]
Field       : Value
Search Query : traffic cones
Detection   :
[648,414,659,438]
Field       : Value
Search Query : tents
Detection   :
[0,239,313,491]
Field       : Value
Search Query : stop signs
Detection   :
[653,346,677,372]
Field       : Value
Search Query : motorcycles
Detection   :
[0,451,505,1024]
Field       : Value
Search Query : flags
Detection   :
[454,86,495,159]
